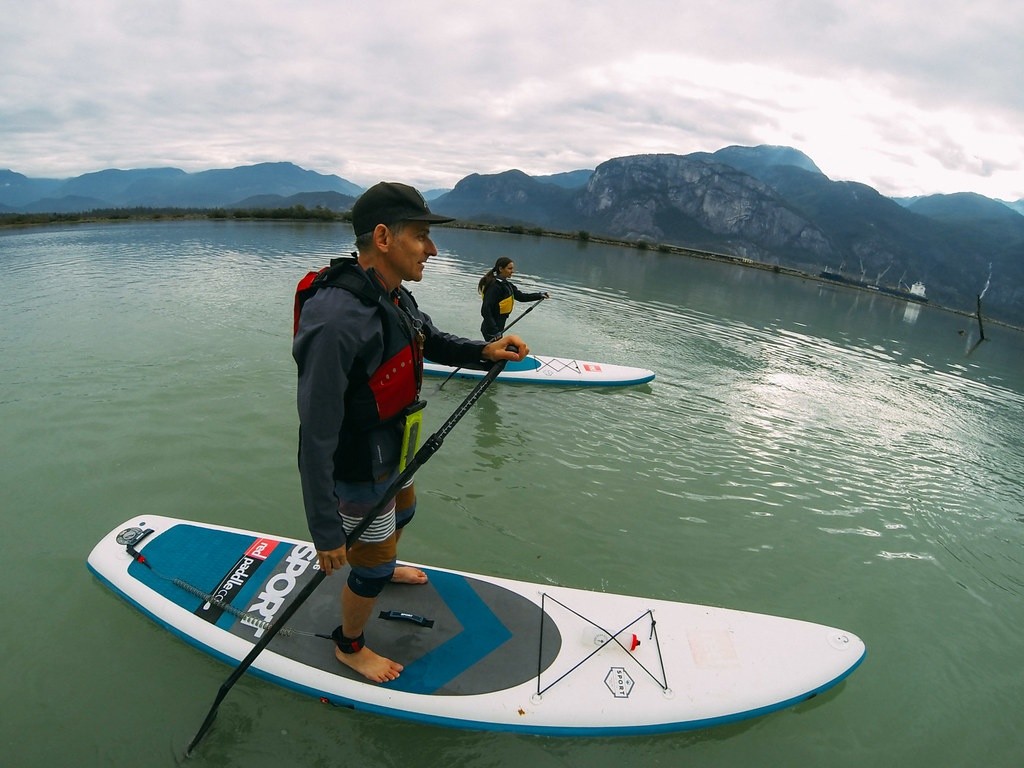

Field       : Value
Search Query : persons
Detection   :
[295,180,529,684]
[478,257,550,363]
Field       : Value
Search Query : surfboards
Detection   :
[86,512,868,738]
[422,354,654,386]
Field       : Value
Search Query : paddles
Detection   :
[188,344,517,755]
[441,292,548,387]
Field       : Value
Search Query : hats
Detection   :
[351,181,456,237]
